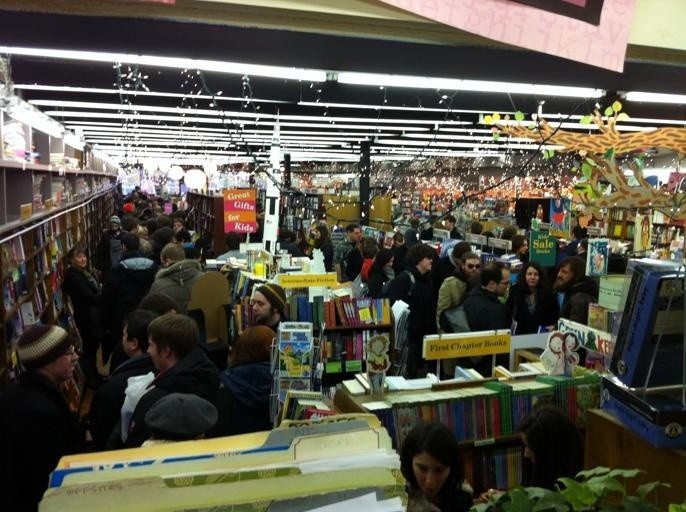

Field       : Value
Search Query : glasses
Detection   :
[468,264,480,269]
[502,281,509,285]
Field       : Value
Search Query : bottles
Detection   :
[246,249,255,271]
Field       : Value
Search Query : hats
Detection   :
[18,324,70,370]
[255,284,286,313]
[145,392,218,434]
[109,215,121,224]
[123,203,134,212]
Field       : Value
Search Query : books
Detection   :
[341,361,599,491]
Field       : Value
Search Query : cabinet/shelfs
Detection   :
[269,338,322,423]
[333,375,585,490]
[259,190,391,255]
[281,305,395,400]
[0,111,122,411]
[584,409,686,512]
[607,206,686,249]
[187,192,224,258]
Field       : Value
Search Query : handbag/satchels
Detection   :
[351,273,370,299]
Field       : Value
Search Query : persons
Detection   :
[304,215,598,377]
[0,186,292,512]
[519,406,586,492]
[397,417,491,512]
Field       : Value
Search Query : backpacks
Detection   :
[382,270,416,298]
